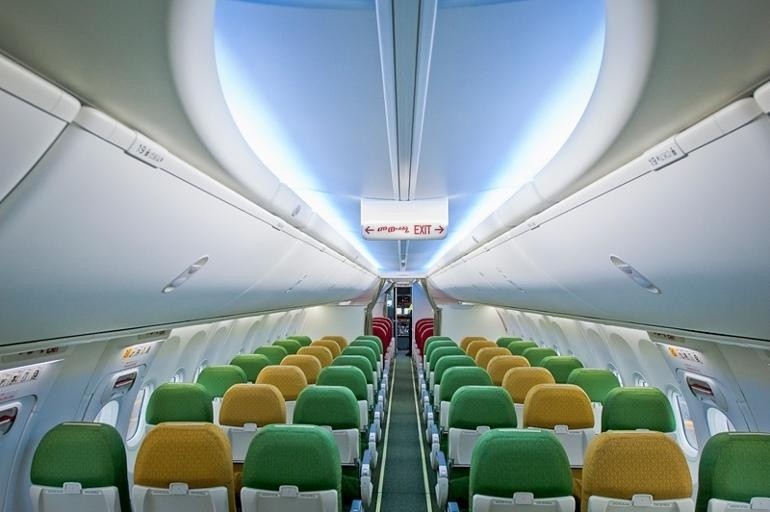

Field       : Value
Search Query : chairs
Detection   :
[240,424,364,512]
[465,340,499,362]
[572,430,694,512]
[523,384,596,495]
[357,335,389,385]
[315,366,379,470]
[601,386,677,443]
[145,382,214,435]
[414,324,434,362]
[430,385,517,470]
[292,385,373,508]
[417,335,453,374]
[539,356,584,383]
[486,355,531,386]
[195,365,248,424]
[447,428,576,511]
[330,355,382,443]
[295,346,333,368]
[474,347,512,372]
[279,354,323,384]
[501,367,556,428]
[459,336,488,354]
[254,365,308,424]
[420,355,479,408]
[507,341,539,355]
[567,368,621,433]
[308,340,341,358]
[426,366,494,443]
[415,328,434,367]
[319,335,348,349]
[418,340,458,383]
[521,347,559,367]
[254,345,287,365]
[496,337,523,348]
[286,335,311,346]
[373,327,387,361]
[229,353,272,383]
[349,339,389,396]
[218,383,287,495]
[418,346,465,398]
[29,421,131,512]
[339,346,385,426]
[132,421,237,512]
[272,340,301,355]
[696,430,770,512]
